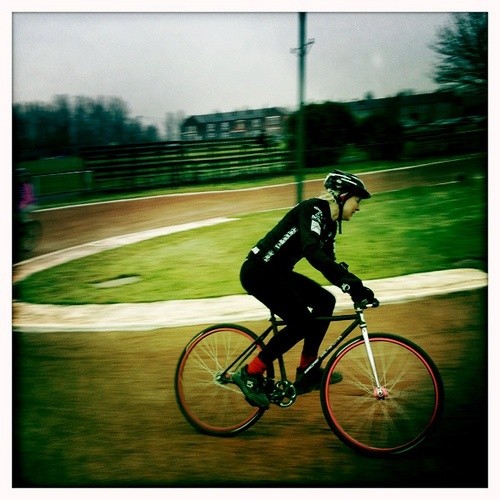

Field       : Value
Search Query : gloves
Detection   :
[326,263,374,303]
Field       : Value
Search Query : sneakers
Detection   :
[232,364,269,408]
[295,365,343,398]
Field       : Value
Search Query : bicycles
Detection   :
[173,285,446,458]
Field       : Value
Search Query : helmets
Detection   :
[323,169,372,200]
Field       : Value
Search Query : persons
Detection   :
[231,170,374,409]
